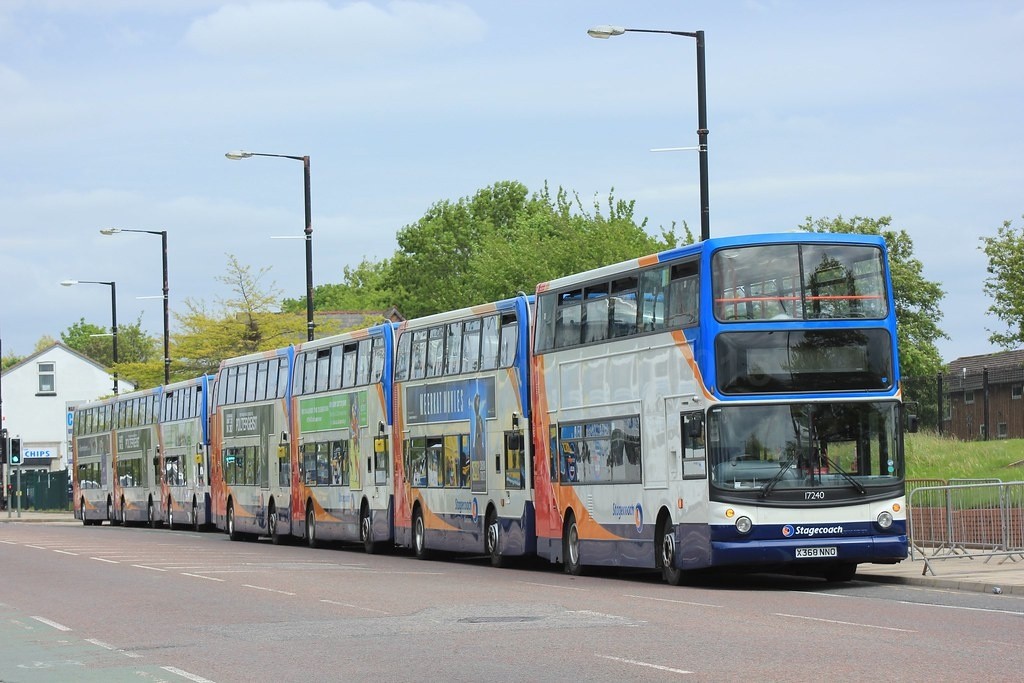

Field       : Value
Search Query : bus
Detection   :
[291,319,402,554]
[210,343,296,545]
[73,373,217,531]
[529,232,909,585]
[393,291,666,568]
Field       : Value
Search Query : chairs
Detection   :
[796,442,830,475]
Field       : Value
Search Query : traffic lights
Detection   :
[8,485,12,490]
[12,439,20,463]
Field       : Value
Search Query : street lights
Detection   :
[225,151,314,342]
[100,227,170,385]
[60,280,118,396]
[588,25,710,242]
